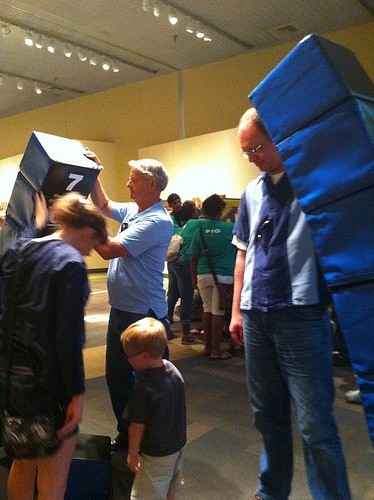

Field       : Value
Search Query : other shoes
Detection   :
[344,389,363,402]
[210,351,232,359]
[181,333,204,345]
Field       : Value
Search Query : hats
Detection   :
[167,193,180,206]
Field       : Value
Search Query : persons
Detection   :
[326,301,364,406]
[227,107,355,500]
[187,194,237,360]
[0,190,111,500]
[162,190,239,241]
[49,145,174,452]
[121,317,188,500]
[166,212,207,344]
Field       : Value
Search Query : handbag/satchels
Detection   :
[216,283,234,310]
[0,394,70,460]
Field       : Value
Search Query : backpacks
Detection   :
[165,234,183,261]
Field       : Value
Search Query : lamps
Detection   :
[0,0,213,95]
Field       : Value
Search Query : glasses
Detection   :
[240,138,268,159]
[121,350,146,360]
